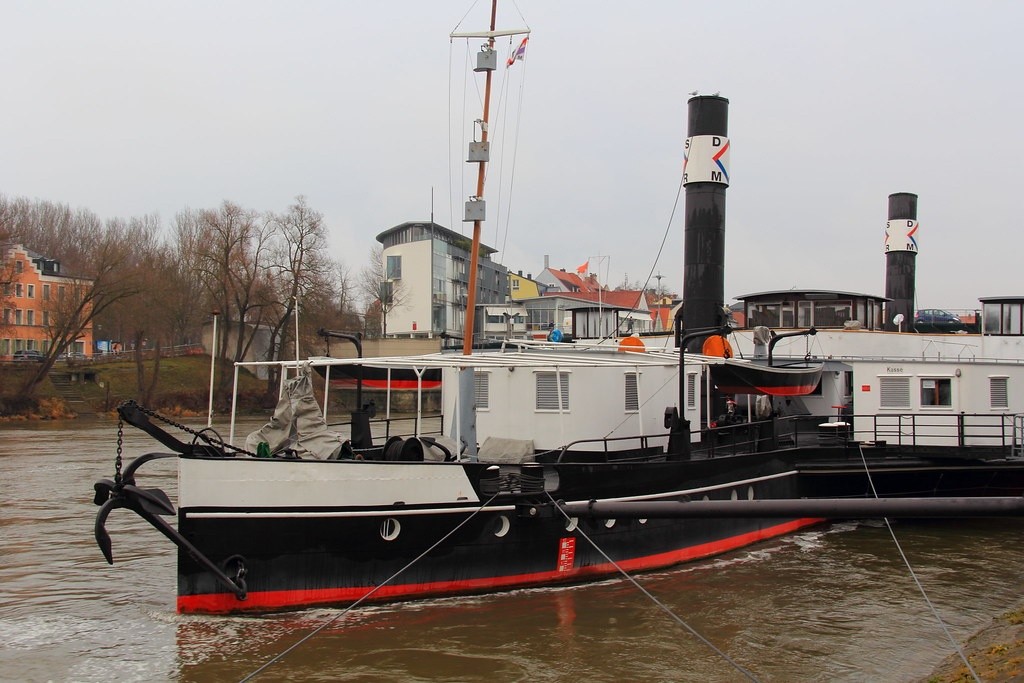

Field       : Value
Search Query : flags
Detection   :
[506,37,528,67]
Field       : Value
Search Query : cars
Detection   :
[58,352,86,359]
[915,309,961,324]
[13,351,45,361]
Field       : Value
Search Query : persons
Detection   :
[626,316,634,334]
[546,320,555,342]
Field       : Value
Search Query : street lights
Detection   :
[505,294,514,338]
[652,274,665,331]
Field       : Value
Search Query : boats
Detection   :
[94,0,1024,619]
